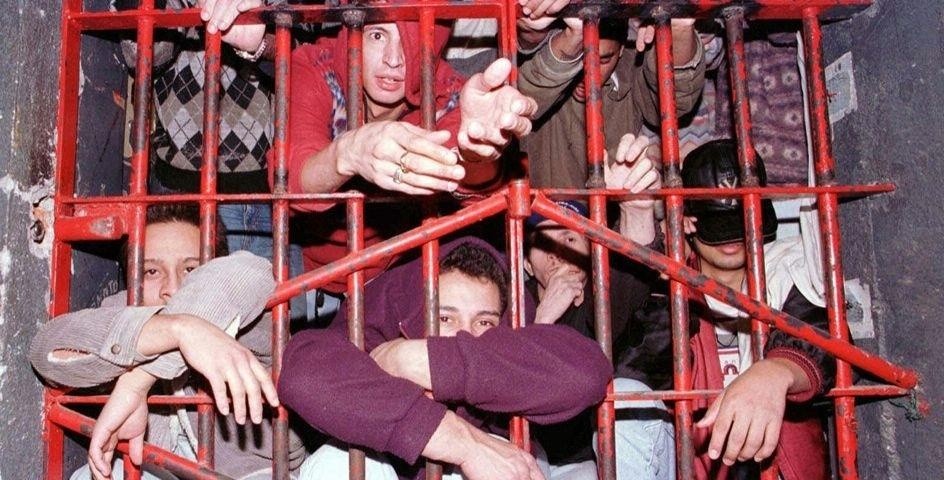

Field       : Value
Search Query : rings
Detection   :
[400,148,409,173]
[393,168,402,183]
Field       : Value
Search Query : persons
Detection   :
[269,19,537,292]
[445,1,570,81]
[276,238,614,480]
[640,14,725,171]
[31,201,305,480]
[661,140,860,480]
[626,24,808,185]
[108,0,307,321]
[513,11,703,189]
[504,202,699,380]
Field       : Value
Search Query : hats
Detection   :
[527,201,591,227]
[681,137,779,247]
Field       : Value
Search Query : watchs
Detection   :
[235,40,267,63]
